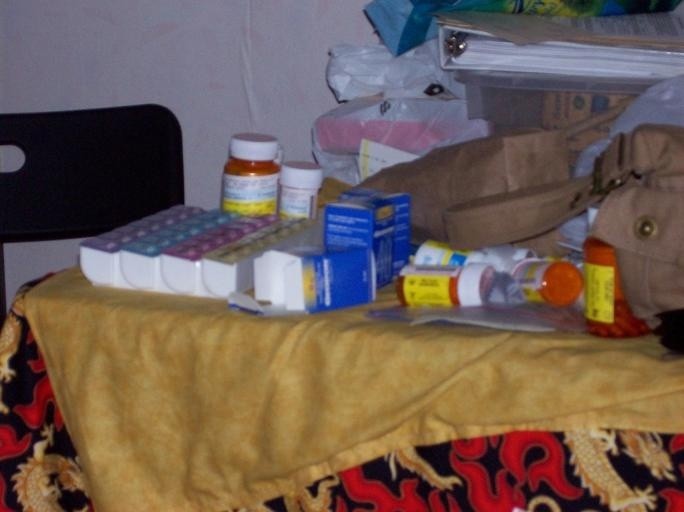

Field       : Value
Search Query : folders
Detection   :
[438,22,684,81]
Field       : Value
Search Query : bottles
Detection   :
[412,239,497,266]
[482,245,537,260]
[397,264,496,308]
[583,203,650,338]
[220,132,279,216]
[508,258,584,308]
[278,162,324,218]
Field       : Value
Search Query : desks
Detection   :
[0,263,684,511]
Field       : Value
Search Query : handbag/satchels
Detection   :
[444,124,684,329]
[354,96,638,257]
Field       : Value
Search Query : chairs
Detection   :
[0,103,184,332]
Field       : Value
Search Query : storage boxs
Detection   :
[453,69,660,131]
